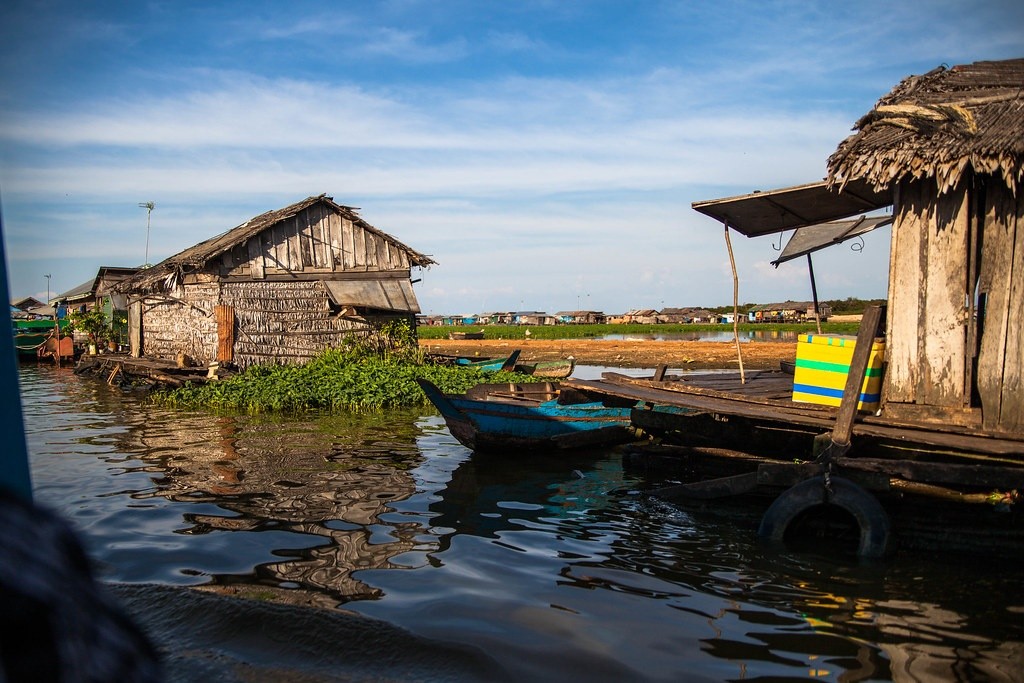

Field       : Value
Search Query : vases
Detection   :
[89,345,97,356]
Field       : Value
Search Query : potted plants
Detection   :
[107,330,120,352]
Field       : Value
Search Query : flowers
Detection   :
[88,333,96,345]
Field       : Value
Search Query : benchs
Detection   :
[487,393,543,403]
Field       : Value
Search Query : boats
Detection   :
[516,358,576,380]
[453,328,486,340]
[416,374,677,454]
[456,349,522,375]
[781,361,795,374]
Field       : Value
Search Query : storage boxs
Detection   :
[793,333,886,413]
